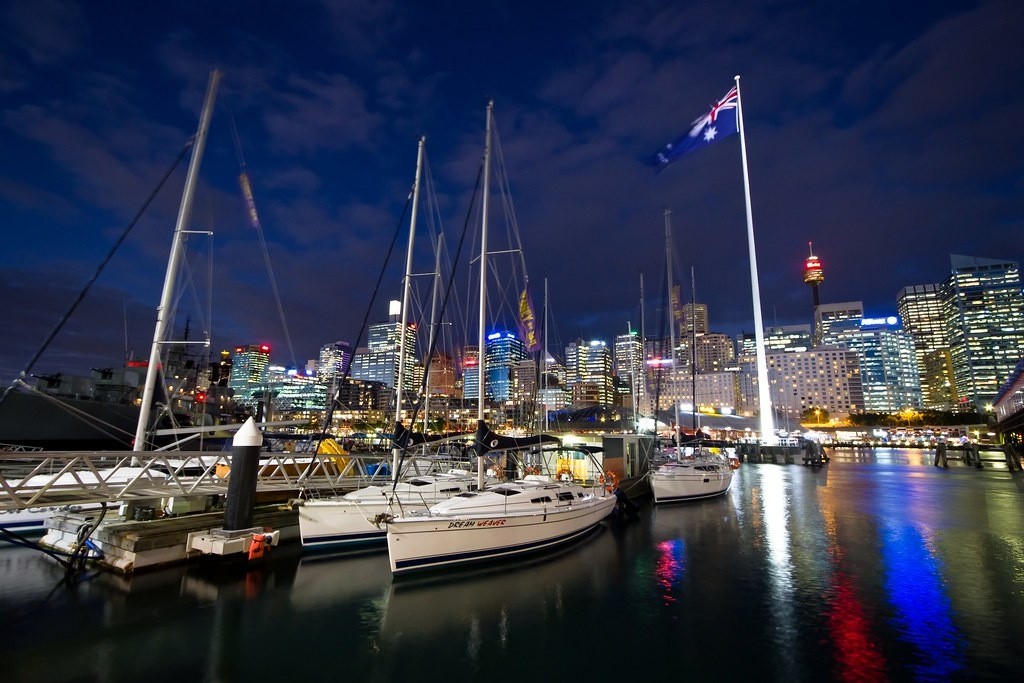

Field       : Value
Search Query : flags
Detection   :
[650,84,738,174]
[518,289,538,354]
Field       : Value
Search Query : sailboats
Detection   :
[0,62,735,585]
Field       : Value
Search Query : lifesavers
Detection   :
[487,465,502,480]
[524,467,539,481]
[600,471,617,493]
[556,469,572,483]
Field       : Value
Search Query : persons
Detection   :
[959,433,971,446]
